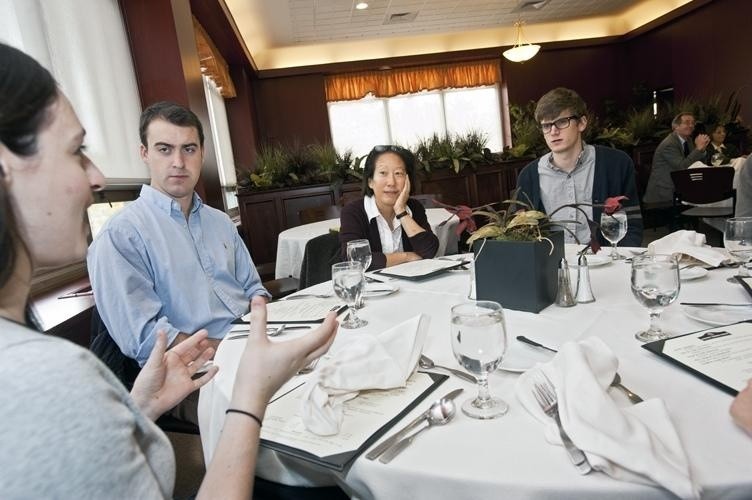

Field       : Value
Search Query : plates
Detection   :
[566,253,611,268]
[495,359,530,376]
[680,307,751,326]
[680,260,707,281]
[362,283,401,297]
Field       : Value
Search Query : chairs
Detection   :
[634,135,751,248]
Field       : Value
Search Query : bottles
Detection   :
[574,254,595,304]
[554,258,577,307]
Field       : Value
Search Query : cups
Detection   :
[710,153,722,167]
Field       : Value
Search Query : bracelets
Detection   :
[225,409,263,429]
[396,209,410,220]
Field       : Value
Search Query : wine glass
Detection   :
[632,254,682,344]
[597,211,626,260]
[724,217,752,283]
[450,300,508,421]
[331,239,373,328]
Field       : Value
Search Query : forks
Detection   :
[532,382,592,474]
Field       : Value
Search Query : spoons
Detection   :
[419,355,477,386]
[228,324,286,342]
[610,373,642,407]
[380,397,457,464]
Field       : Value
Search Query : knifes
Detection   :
[364,388,465,461]
[231,325,312,332]
[367,289,395,294]
[679,301,751,311]
[516,336,555,355]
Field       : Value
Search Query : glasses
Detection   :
[536,116,576,134]
[374,144,405,154]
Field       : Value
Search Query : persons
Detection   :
[705,124,742,165]
[505,85,645,249]
[640,112,725,249]
[86,100,274,430]
[0,41,340,500]
[338,143,440,272]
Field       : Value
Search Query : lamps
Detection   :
[503,0,542,65]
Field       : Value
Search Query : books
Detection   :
[641,318,752,398]
[733,274,752,299]
[229,296,348,326]
[256,369,451,474]
[372,255,471,282]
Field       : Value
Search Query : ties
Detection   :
[684,141,690,158]
